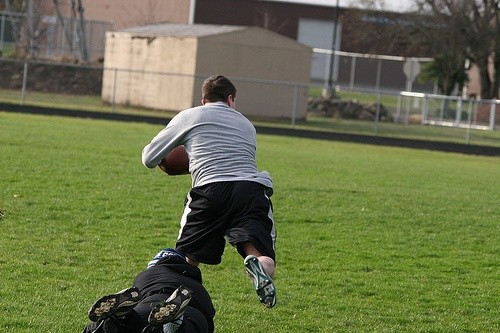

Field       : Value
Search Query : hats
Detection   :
[147,248,186,268]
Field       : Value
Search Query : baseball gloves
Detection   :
[159,145,189,175]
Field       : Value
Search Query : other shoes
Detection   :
[148,285,193,327]
[87,286,141,322]
[244,255,276,308]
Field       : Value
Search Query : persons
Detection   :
[141,74,278,309]
[82,248,216,332]
